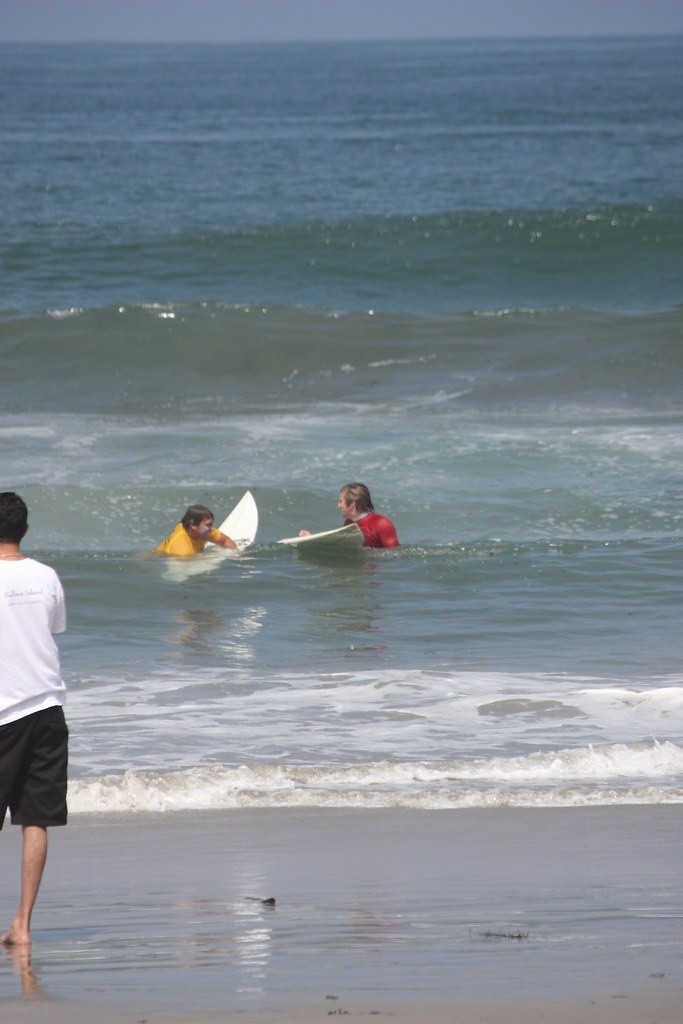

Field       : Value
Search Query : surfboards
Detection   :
[278,521,364,546]
[204,490,259,548]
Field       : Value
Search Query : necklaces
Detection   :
[0,554,23,557]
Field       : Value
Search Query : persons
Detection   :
[298,483,399,549]
[0,492,69,946]
[155,505,237,556]
